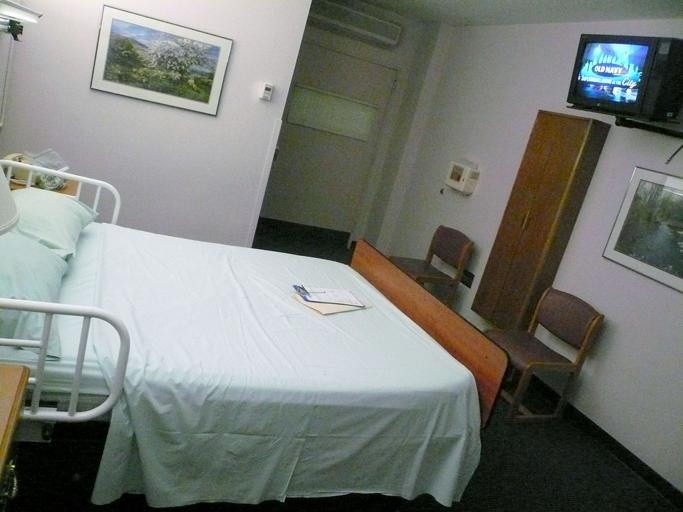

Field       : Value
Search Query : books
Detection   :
[293,284,366,315]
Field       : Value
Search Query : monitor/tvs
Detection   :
[566,34,683,122]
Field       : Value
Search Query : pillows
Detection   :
[0,232,69,359]
[11,189,101,259]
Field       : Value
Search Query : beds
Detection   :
[0,160,510,511]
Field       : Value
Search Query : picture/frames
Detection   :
[601,165,683,293]
[90,4,234,116]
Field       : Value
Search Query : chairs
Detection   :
[387,225,474,304]
[484,286,605,425]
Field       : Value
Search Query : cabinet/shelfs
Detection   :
[470,109,611,330]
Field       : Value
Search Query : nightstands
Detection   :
[1,364,30,512]
